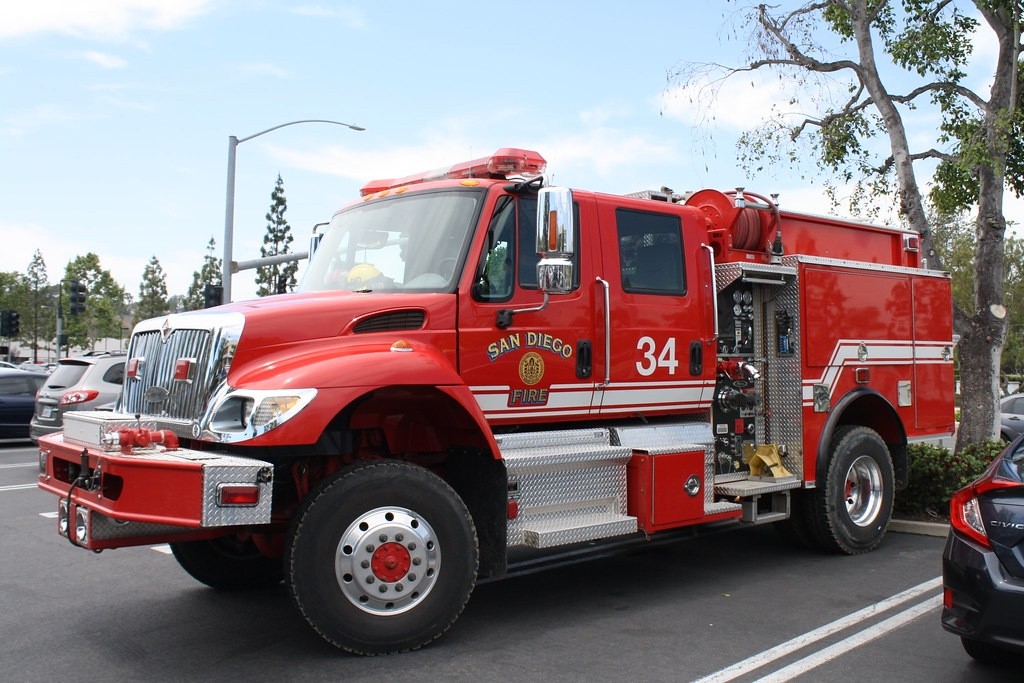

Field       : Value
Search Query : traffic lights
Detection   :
[7,310,21,339]
[71,283,87,316]
[222,119,367,306]
[199,284,223,310]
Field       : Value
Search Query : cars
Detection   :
[0,367,53,440]
[999,393,1024,447]
[940,430,1024,663]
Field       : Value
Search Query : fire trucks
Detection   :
[35,149,952,659]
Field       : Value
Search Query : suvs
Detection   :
[29,350,128,447]
